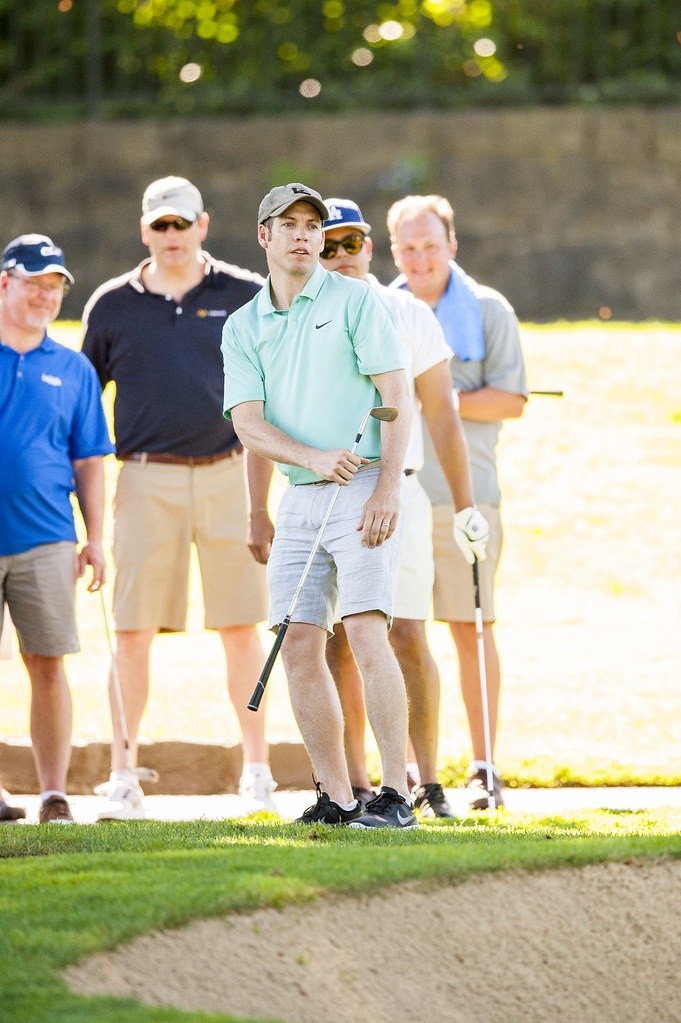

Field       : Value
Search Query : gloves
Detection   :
[420,389,459,413]
[453,504,489,565]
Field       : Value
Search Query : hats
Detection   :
[258,182,329,223]
[321,198,371,235]
[0,233,75,285]
[140,175,204,225]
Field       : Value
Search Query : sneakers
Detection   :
[352,783,453,818]
[94,767,160,822]
[467,760,503,809]
[39,795,74,823]
[0,789,26,820]
[238,762,278,817]
[349,785,419,831]
[295,773,363,828]
[406,763,421,809]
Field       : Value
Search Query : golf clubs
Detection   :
[458,508,498,824]
[98,587,160,787]
[244,405,399,712]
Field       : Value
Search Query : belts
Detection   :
[116,444,244,467]
[296,459,380,485]
[405,469,414,476]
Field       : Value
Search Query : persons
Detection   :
[221,184,528,827]
[76,176,277,819]
[0,234,105,821]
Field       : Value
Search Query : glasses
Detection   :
[320,234,367,259]
[393,238,450,257]
[7,272,69,297]
[151,219,197,231]
